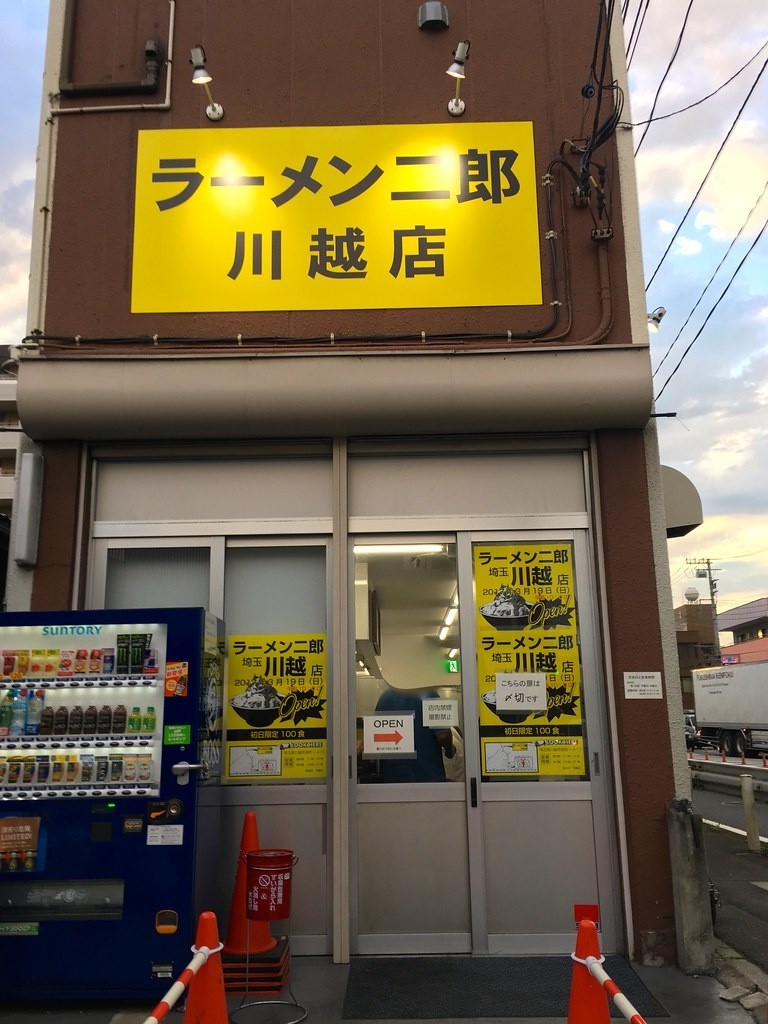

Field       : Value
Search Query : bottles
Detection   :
[0,644,158,746]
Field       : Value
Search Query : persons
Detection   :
[374,687,456,782]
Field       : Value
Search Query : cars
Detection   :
[683,708,720,751]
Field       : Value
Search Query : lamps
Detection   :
[446,40,471,114]
[190,44,224,121]
[647,306,667,333]
[438,588,459,640]
[448,649,457,659]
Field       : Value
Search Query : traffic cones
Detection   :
[209,810,291,999]
[567,919,612,1024]
[182,912,230,1024]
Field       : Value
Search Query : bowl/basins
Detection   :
[478,602,535,631]
[482,690,531,724]
[229,695,288,727]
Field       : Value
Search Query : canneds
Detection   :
[0,849,37,873]
[74,649,115,675]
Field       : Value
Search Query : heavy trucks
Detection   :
[692,659,768,758]
